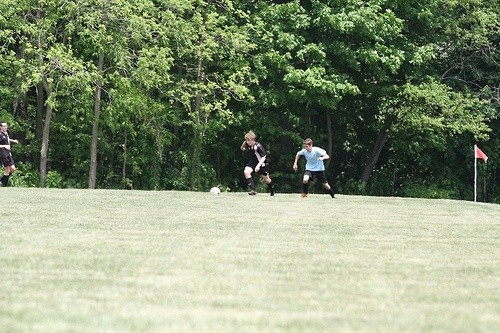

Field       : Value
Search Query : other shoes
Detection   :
[0,174,10,187]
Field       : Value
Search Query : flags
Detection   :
[477,147,488,164]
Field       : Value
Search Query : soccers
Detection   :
[209,188,221,195]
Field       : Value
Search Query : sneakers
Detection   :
[331,195,340,200]
[248,190,255,196]
[299,192,308,200]
[270,188,275,196]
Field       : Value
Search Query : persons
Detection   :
[0,122,18,187]
[293,138,339,199]
[240,130,275,196]
